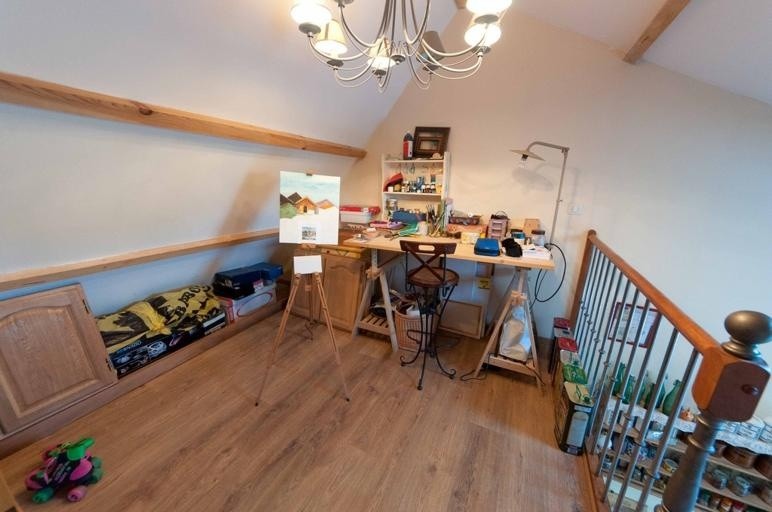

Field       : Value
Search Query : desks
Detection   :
[342,229,557,397]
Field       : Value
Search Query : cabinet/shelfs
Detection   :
[0,281,122,435]
[379,151,451,229]
[593,395,772,512]
[289,229,374,334]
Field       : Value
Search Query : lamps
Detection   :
[510,140,570,252]
[290,1,515,95]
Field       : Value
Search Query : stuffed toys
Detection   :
[24,436,102,504]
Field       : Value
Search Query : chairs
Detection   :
[398,240,461,390]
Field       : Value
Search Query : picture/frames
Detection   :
[411,126,451,159]
[415,136,443,155]
[607,301,661,348]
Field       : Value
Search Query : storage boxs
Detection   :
[108,262,285,379]
[339,210,371,224]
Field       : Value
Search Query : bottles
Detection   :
[402,130,413,159]
[530,228,546,247]
[387,179,443,195]
[610,361,681,417]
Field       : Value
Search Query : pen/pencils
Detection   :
[427,204,445,237]
